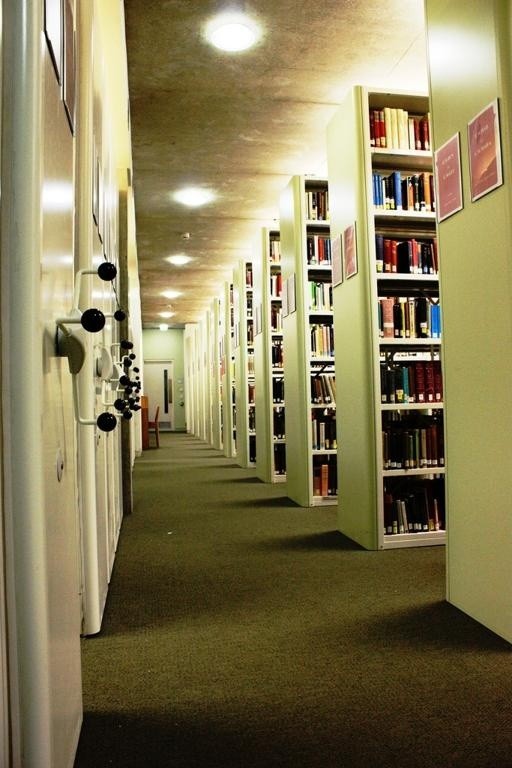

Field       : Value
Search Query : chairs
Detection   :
[145,406,162,447]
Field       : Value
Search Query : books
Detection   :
[370,107,444,535]
[305,190,337,497]
[246,268,255,462]
[269,240,286,475]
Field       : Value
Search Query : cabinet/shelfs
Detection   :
[323,84,445,553]
[183,226,286,485]
[277,173,339,507]
[421,2,511,646]
